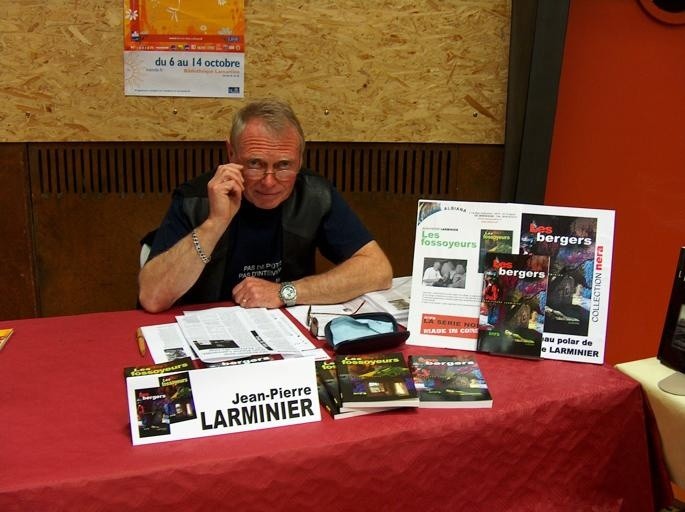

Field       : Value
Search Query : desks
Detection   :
[613,356,685,503]
[1,300,673,512]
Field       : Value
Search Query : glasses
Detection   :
[307,300,367,338]
[240,152,302,182]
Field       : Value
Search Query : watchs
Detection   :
[280,279,299,308]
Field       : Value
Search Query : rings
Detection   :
[242,297,247,302]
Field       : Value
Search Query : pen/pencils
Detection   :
[136,328,145,356]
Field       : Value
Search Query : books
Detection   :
[406,197,617,365]
[141,276,412,363]
[1,327,13,348]
[123,350,495,447]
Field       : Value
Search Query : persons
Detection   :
[137,100,394,314]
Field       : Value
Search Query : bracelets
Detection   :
[192,230,212,264]
